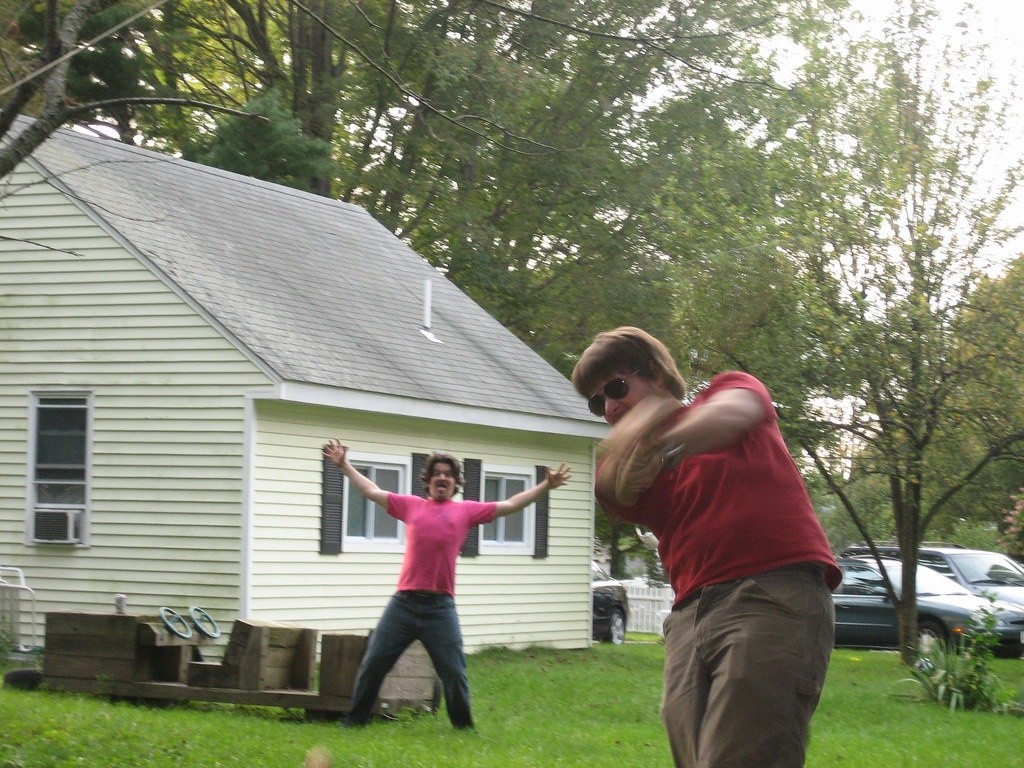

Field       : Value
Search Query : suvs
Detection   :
[841,540,1024,613]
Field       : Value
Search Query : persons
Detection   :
[570,324,844,768]
[322,436,572,732]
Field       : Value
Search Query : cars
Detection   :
[590,562,632,646]
[831,556,1024,662]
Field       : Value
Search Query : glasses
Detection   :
[588,370,641,417]
